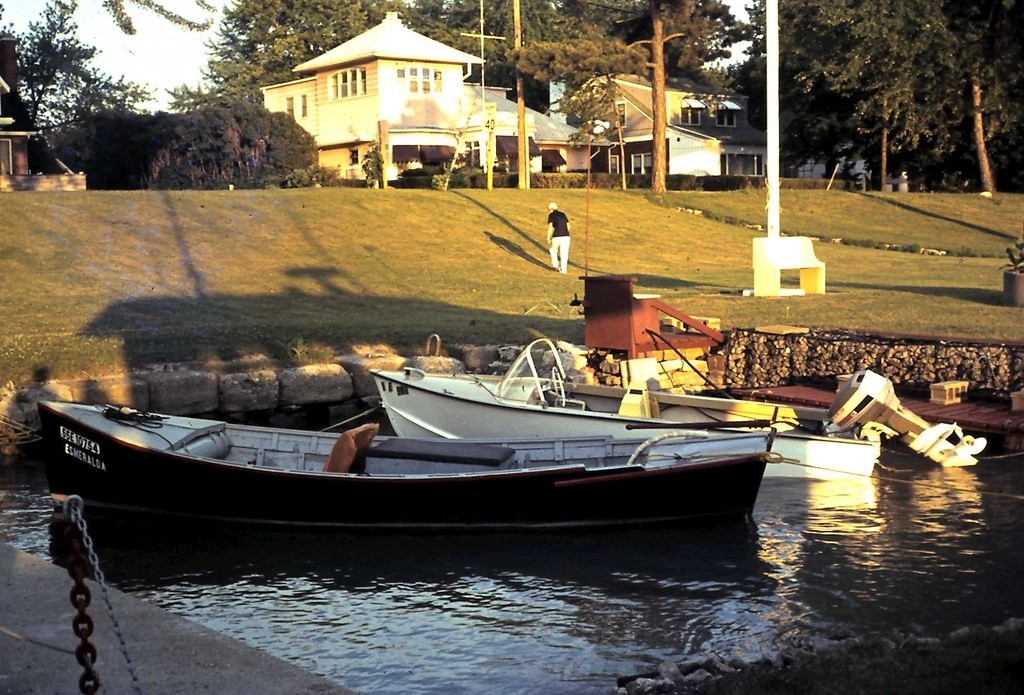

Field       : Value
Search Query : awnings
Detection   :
[421,146,457,162]
[393,145,419,164]
[681,98,708,110]
[542,149,567,167]
[496,137,543,157]
[717,100,745,112]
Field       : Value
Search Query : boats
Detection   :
[371,367,988,486]
[37,399,777,533]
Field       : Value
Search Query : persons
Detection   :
[546,202,571,274]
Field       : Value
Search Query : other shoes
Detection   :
[558,270,567,274]
[550,267,559,271]
